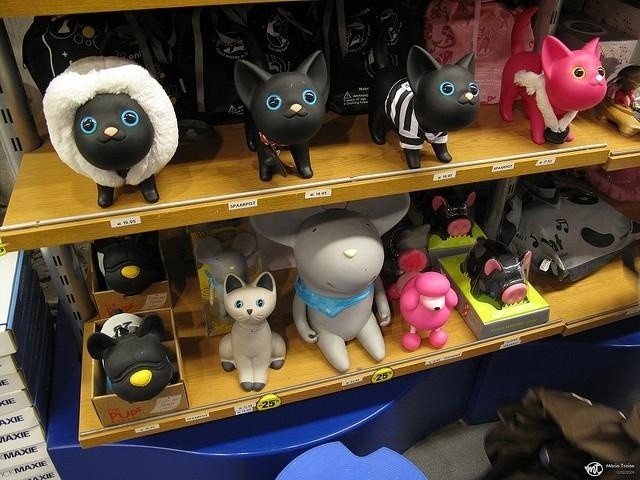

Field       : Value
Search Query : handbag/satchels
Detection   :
[484,386,640,480]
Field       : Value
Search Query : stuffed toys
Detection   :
[86,182,532,403]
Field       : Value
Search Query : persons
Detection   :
[597,65,640,137]
[367,44,480,170]
[41,51,181,209]
[496,5,608,144]
[233,49,331,183]
[86,182,532,403]
